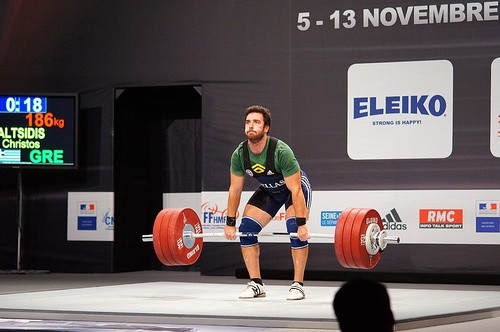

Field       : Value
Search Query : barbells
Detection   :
[142,207,400,269]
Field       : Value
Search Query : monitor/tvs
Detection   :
[0,92,79,170]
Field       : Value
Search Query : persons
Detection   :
[333,278,395,332]
[226,106,312,300]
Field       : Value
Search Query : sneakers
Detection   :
[286,282,305,299]
[239,281,266,298]
